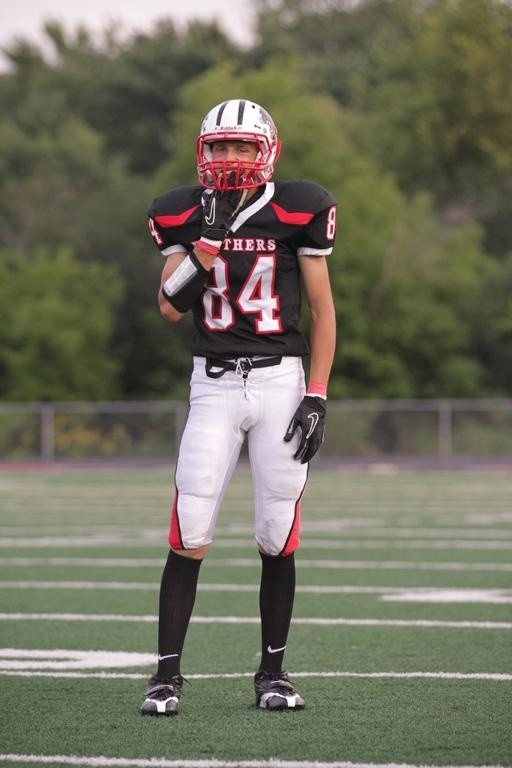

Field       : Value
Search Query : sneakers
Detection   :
[140,673,182,716]
[255,670,306,710]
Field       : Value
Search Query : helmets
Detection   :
[201,97,280,182]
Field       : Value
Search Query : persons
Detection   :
[140,97,338,715]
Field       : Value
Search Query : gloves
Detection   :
[197,172,246,257]
[284,382,328,464]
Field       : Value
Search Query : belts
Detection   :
[206,355,282,378]
